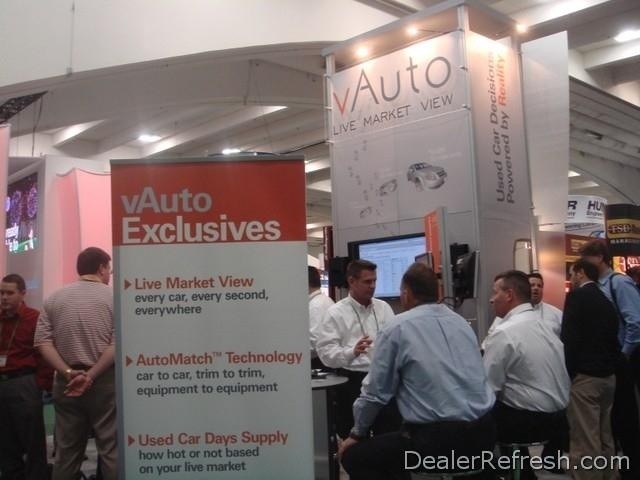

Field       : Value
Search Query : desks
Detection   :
[311,368,349,480]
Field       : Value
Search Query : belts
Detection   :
[0,368,37,381]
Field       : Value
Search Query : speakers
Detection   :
[451,243,469,272]
[328,256,349,289]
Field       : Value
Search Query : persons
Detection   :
[32,246,119,480]
[308,238,640,480]
[0,274,48,480]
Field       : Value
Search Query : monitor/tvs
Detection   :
[351,232,426,301]
[415,251,433,271]
[453,249,480,299]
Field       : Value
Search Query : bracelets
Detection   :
[65,369,72,380]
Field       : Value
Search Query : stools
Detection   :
[495,438,549,480]
[412,466,483,480]
